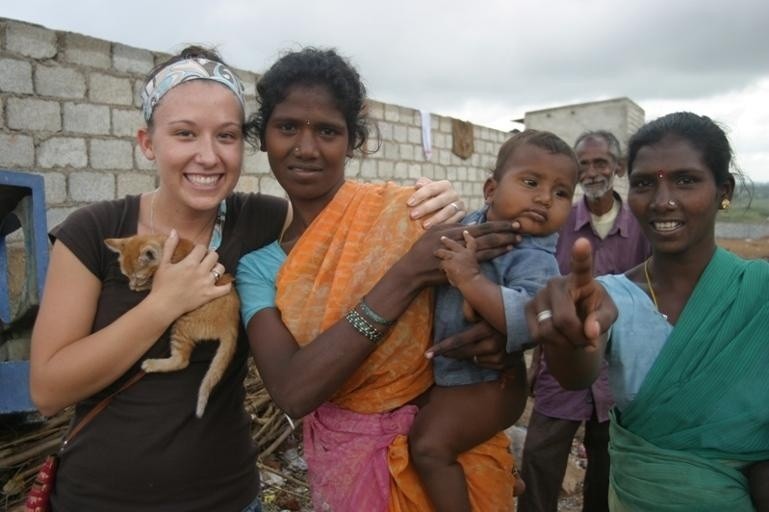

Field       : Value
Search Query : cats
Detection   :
[101,232,244,421]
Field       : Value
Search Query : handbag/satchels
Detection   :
[27,454,58,512]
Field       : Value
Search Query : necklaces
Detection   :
[146,189,221,253]
[644,259,671,322]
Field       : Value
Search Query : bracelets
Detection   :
[358,298,396,328]
[345,312,387,346]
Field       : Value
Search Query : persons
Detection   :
[229,41,523,511]
[28,44,469,511]
[402,127,577,510]
[514,129,653,511]
[522,106,767,511]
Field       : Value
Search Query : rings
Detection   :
[450,201,460,213]
[210,270,222,281]
[537,310,551,325]
